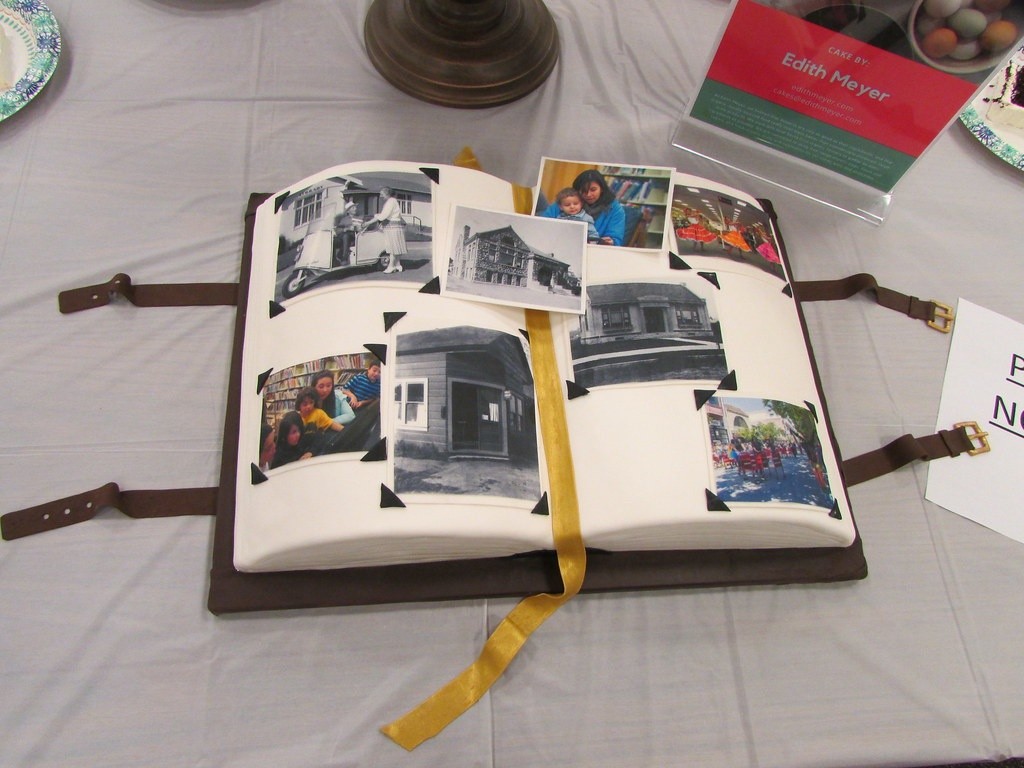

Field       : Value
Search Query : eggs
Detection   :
[916,0,1020,62]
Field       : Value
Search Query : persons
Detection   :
[334,186,409,274]
[540,169,626,246]
[257,359,381,475]
[710,421,829,498]
[675,207,781,274]
